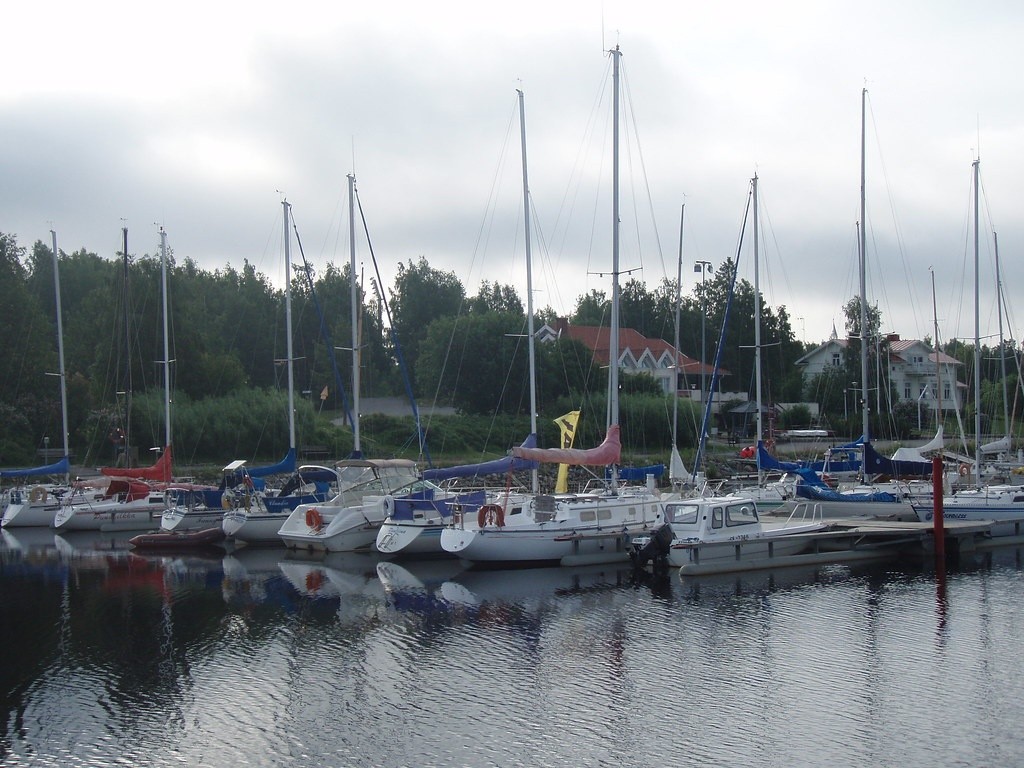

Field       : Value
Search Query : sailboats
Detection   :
[0,17,1024,574]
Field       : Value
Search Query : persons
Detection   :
[116,447,131,468]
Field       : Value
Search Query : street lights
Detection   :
[851,381,860,439]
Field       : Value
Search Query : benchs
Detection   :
[304,506,342,523]
[299,445,333,460]
[36,449,78,465]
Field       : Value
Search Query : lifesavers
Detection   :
[31,486,47,503]
[306,570,321,594]
[306,510,321,530]
[221,490,235,510]
[818,475,832,488]
[478,504,505,527]
[960,463,971,477]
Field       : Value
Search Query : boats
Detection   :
[128,525,224,549]
[630,494,829,568]
